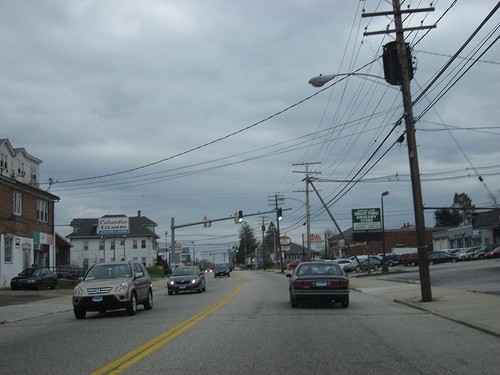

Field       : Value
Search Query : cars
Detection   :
[10,267,58,291]
[56,264,85,278]
[286,261,351,307]
[166,265,204,295]
[323,245,500,272]
[214,262,230,278]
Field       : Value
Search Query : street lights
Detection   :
[381,189,390,275]
[308,73,434,305]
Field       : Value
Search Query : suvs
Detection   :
[71,260,154,320]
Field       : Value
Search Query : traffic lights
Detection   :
[239,210,242,223]
[276,208,283,221]
